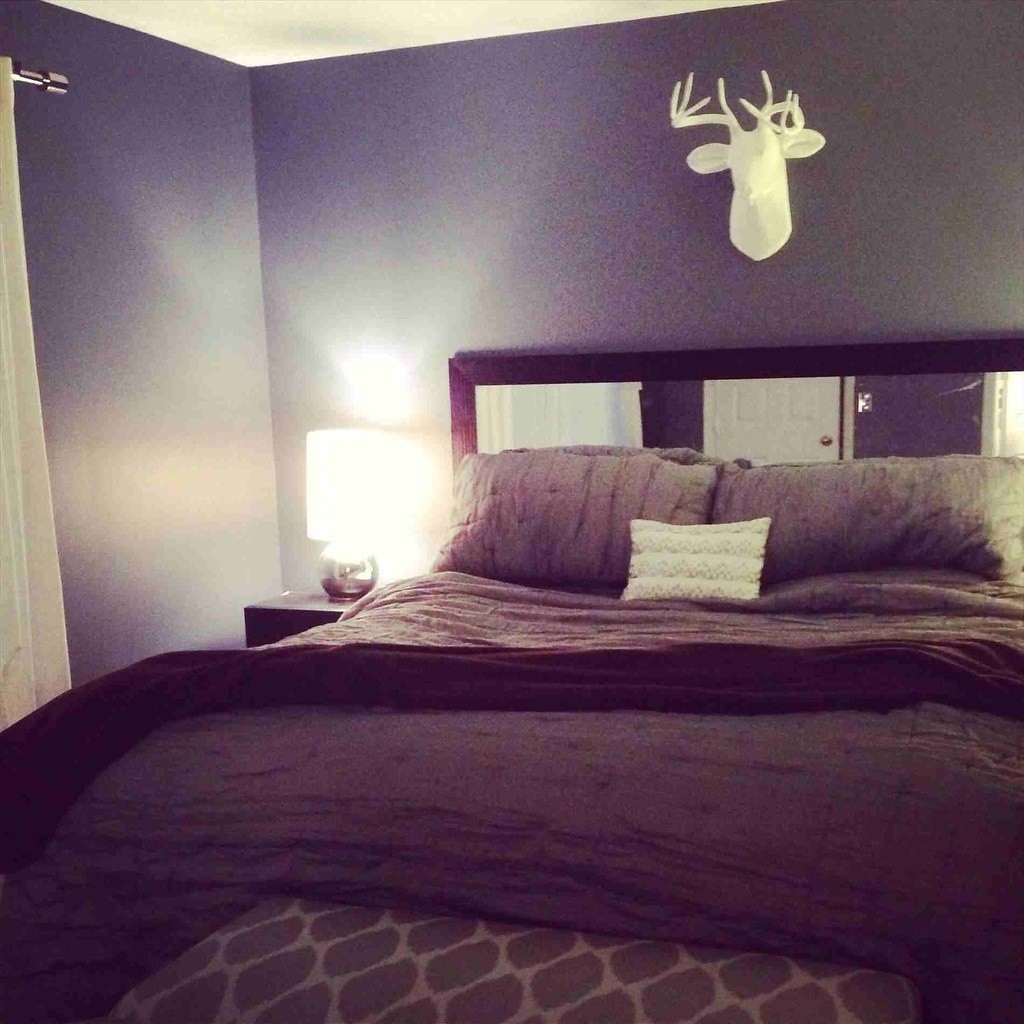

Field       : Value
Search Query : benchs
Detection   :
[107,893,925,1024]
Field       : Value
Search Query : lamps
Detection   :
[306,429,395,598]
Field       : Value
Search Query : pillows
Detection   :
[431,453,719,588]
[620,513,771,600]
[710,454,1024,584]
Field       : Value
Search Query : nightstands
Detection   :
[244,589,358,649]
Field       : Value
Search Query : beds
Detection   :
[0,338,1024,1024]
